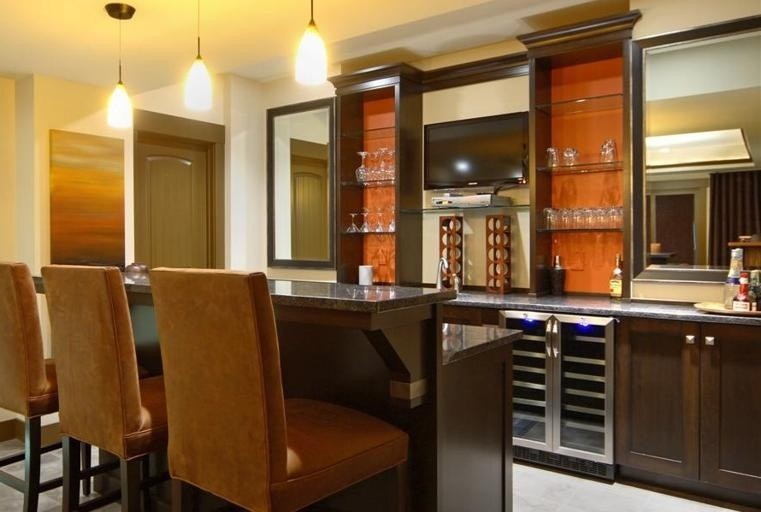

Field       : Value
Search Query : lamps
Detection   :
[294,0,327,87]
[182,0,213,110]
[105,3,135,125]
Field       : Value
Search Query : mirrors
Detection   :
[632,15,760,284]
[266,96,336,268]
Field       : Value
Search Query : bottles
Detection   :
[724,248,761,311]
[549,255,566,296]
[608,254,622,298]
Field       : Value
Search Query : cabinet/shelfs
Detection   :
[614,317,761,511]
[515,10,633,299]
[328,62,422,287]
[499,310,614,484]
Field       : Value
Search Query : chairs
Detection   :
[149,265,410,511]
[40,264,171,511]
[0,261,92,512]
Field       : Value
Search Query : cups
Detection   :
[542,206,623,230]
[562,147,580,171]
[359,266,373,286]
[545,146,560,173]
[599,137,617,169]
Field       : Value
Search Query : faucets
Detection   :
[437,256,449,288]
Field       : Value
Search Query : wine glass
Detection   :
[355,148,396,186]
[346,205,396,233]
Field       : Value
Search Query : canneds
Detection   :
[748,270,761,301]
